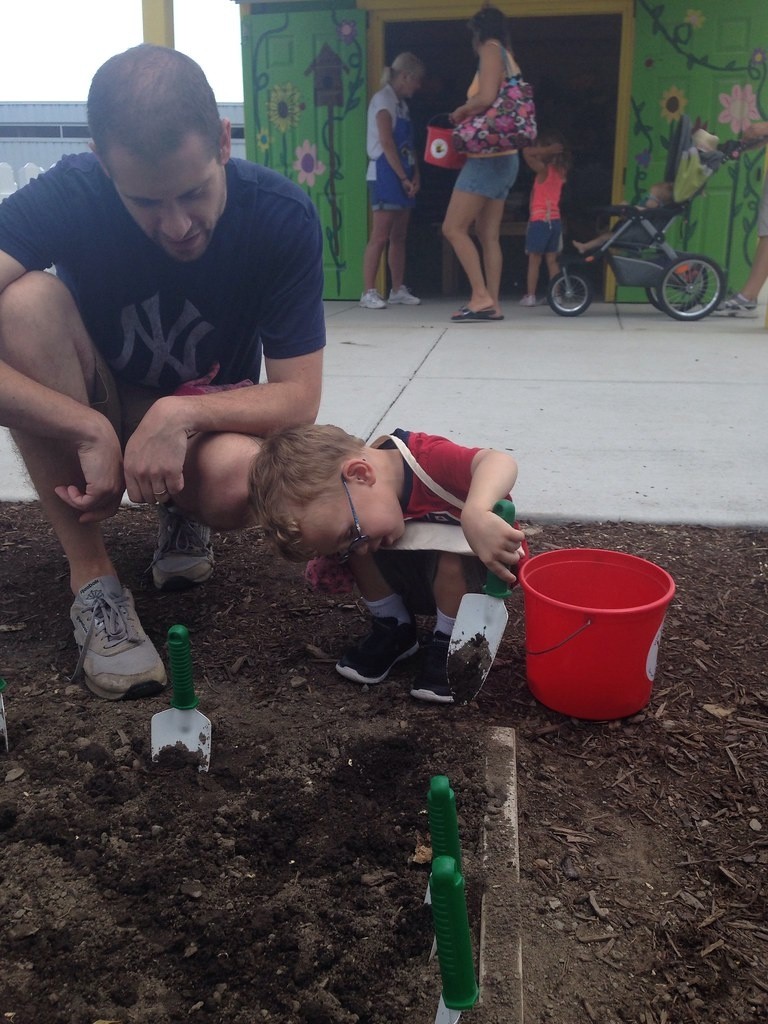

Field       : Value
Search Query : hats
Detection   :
[691,128,720,155]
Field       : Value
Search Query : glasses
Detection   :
[336,459,370,566]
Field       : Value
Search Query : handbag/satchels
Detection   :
[452,43,536,155]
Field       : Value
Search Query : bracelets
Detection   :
[402,177,407,182]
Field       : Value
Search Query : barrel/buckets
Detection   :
[423,112,466,170]
[517,548,675,719]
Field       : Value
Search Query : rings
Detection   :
[154,489,168,498]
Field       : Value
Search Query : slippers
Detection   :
[451,306,496,319]
[473,315,504,320]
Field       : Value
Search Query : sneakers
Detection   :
[334,617,419,684]
[144,503,215,589]
[358,288,386,309]
[69,574,168,700]
[709,292,758,318]
[520,295,536,306]
[541,295,562,305]
[388,284,420,305]
[410,630,454,704]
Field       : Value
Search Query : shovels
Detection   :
[428,856,479,1024]
[447,499,515,707]
[422,775,464,965]
[151,624,212,773]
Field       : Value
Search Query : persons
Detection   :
[359,51,422,308]
[714,121,768,317]
[520,134,571,306]
[572,183,673,253]
[441,8,519,320]
[247,417,530,702]
[0,44,327,700]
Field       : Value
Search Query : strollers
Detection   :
[544,113,766,322]
